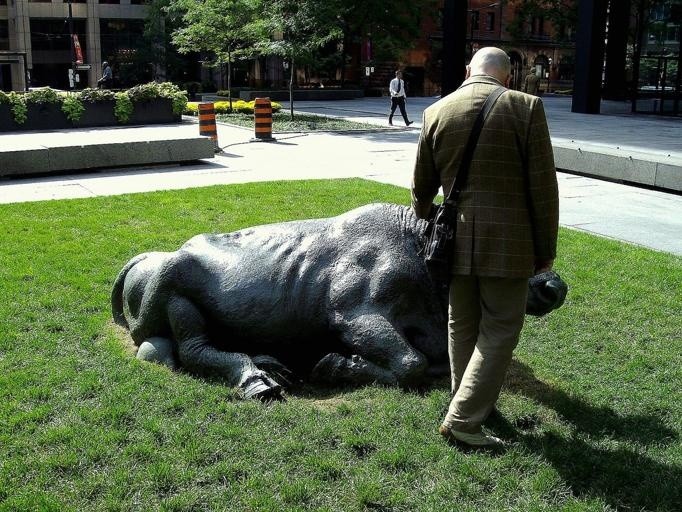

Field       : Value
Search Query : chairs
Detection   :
[98,63,117,88]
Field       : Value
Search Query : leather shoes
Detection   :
[406,121,414,126]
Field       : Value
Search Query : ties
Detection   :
[396,79,402,95]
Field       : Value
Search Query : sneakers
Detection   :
[437,419,507,457]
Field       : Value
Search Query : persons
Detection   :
[412,45,560,454]
[388,70,413,126]
[524,67,540,95]
[97,60,113,89]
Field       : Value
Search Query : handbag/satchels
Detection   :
[420,200,455,296]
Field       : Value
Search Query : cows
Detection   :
[109,201,569,401]
